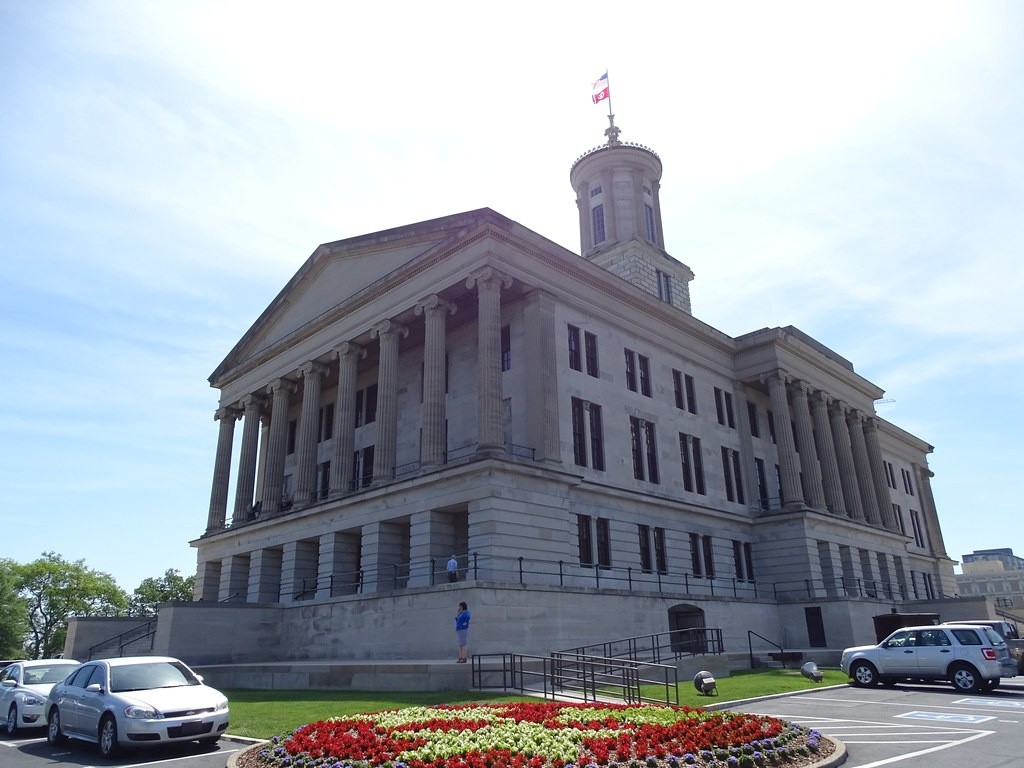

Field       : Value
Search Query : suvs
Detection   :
[840,625,1018,693]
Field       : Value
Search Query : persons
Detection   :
[246,500,262,518]
[455,601,470,663]
[446,554,459,583]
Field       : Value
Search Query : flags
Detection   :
[592,73,610,104]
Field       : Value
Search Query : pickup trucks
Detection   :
[940,620,1024,675]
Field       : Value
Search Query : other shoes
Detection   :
[456,657,467,663]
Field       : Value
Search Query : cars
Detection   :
[0,658,84,735]
[44,656,231,760]
